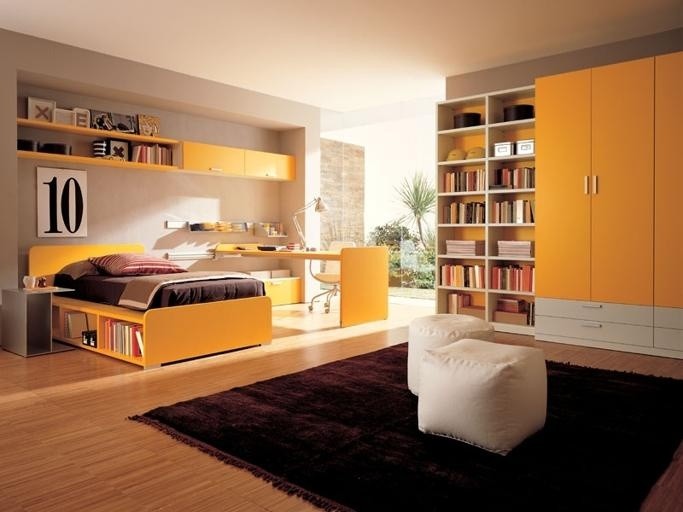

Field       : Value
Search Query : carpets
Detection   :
[127,339,683,512]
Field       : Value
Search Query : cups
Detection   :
[23,276,35,289]
[92,140,107,157]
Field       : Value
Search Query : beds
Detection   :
[28,245,272,370]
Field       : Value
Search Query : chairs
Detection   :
[309,240,356,313]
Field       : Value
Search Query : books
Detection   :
[64,311,143,359]
[131,144,174,165]
[440,167,535,328]
[236,222,284,250]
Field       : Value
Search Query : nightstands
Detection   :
[0,286,76,358]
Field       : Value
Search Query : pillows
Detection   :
[89,253,188,277]
[57,253,101,280]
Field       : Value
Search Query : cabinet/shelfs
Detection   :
[172,141,296,183]
[17,118,178,172]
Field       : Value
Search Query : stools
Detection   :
[419,339,548,456]
[408,314,492,397]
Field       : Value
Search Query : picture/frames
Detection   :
[27,96,91,128]
[107,138,131,162]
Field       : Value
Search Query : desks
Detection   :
[216,242,389,328]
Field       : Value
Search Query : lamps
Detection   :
[292,197,329,251]
[167,254,215,261]
